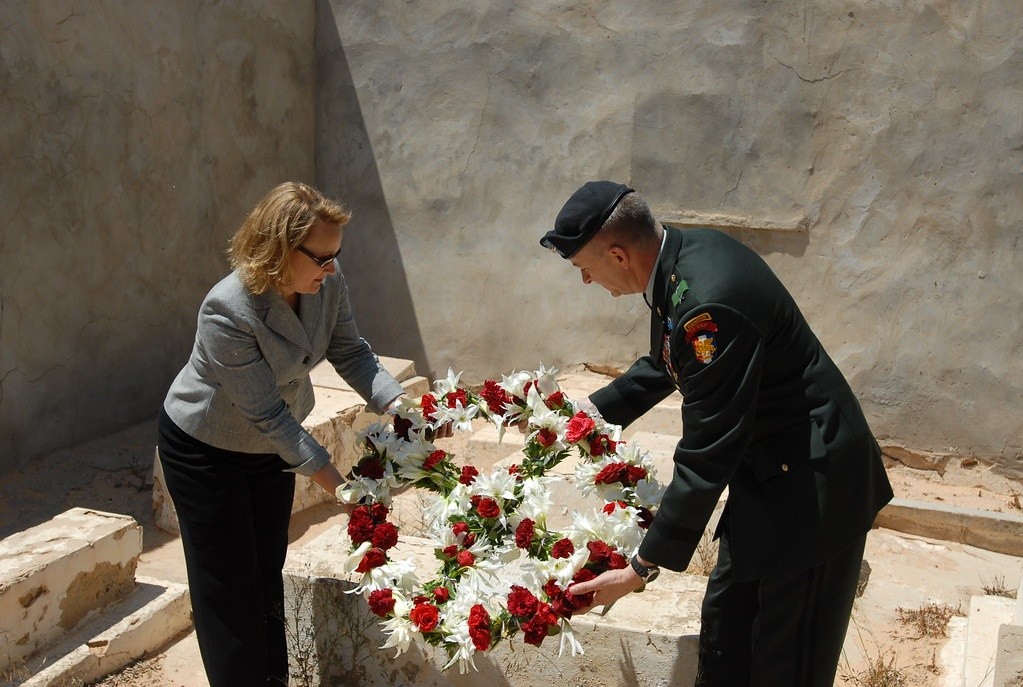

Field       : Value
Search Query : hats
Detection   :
[539,180,636,260]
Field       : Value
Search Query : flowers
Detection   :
[334,361,666,674]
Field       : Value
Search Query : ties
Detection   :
[642,292,652,311]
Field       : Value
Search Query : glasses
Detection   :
[297,244,342,268]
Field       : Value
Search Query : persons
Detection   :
[157,181,456,687]
[539,180,895,687]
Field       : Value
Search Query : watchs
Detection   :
[631,554,661,584]
